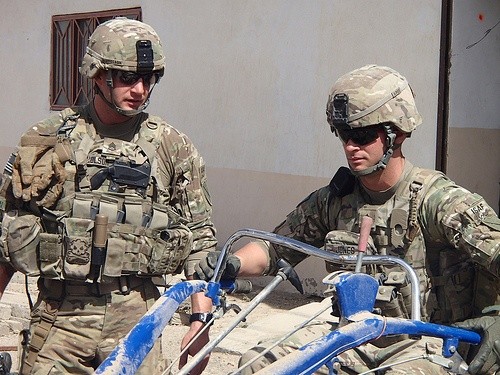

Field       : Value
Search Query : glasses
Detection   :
[119,71,160,86]
[336,125,383,145]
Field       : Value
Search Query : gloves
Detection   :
[193,250,241,280]
[452,305,500,375]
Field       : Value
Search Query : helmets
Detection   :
[79,17,166,79]
[325,64,423,133]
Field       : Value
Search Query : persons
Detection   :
[0,16,217,375]
[193,64,500,375]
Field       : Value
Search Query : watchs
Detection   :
[190,313,214,326]
